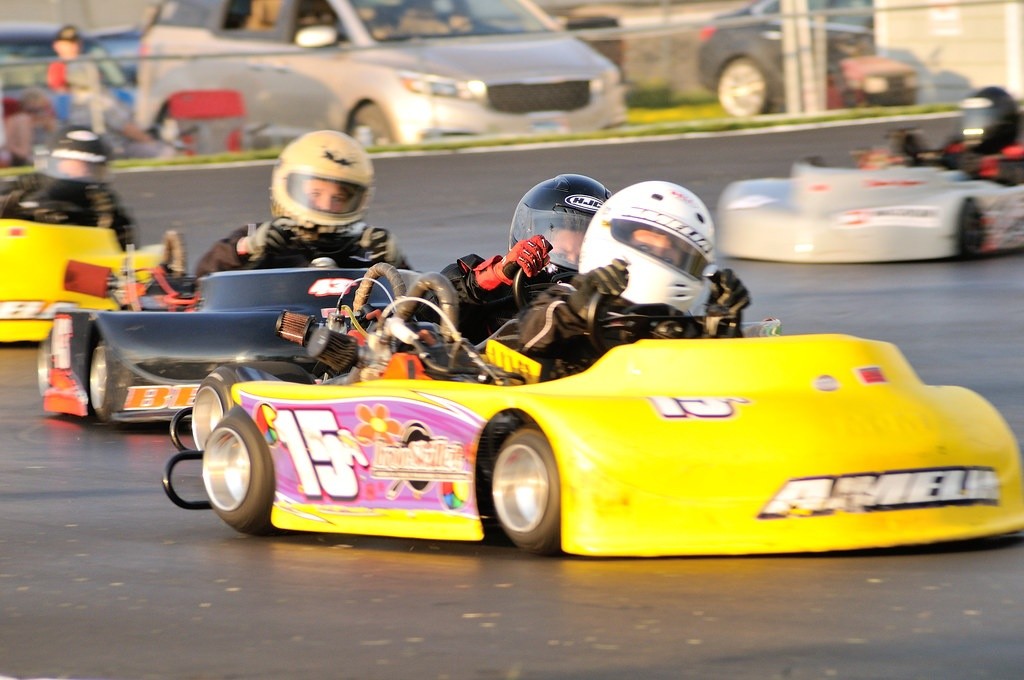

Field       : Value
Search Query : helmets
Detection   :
[507,174,612,276]
[40,126,108,187]
[578,180,714,313]
[958,85,1019,156]
[271,130,374,241]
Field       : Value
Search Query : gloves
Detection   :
[11,174,46,201]
[472,235,554,290]
[566,258,629,322]
[887,129,920,158]
[86,184,119,221]
[238,216,296,261]
[707,268,750,335]
[943,151,980,174]
[359,226,396,266]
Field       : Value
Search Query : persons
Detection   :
[414,173,751,357]
[48,28,158,151]
[0,96,136,249]
[195,130,411,275]
[944,87,1024,186]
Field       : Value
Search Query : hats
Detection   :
[54,25,85,43]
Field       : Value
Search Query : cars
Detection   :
[694,1,875,117]
[136,1,628,151]
[0,22,136,170]
